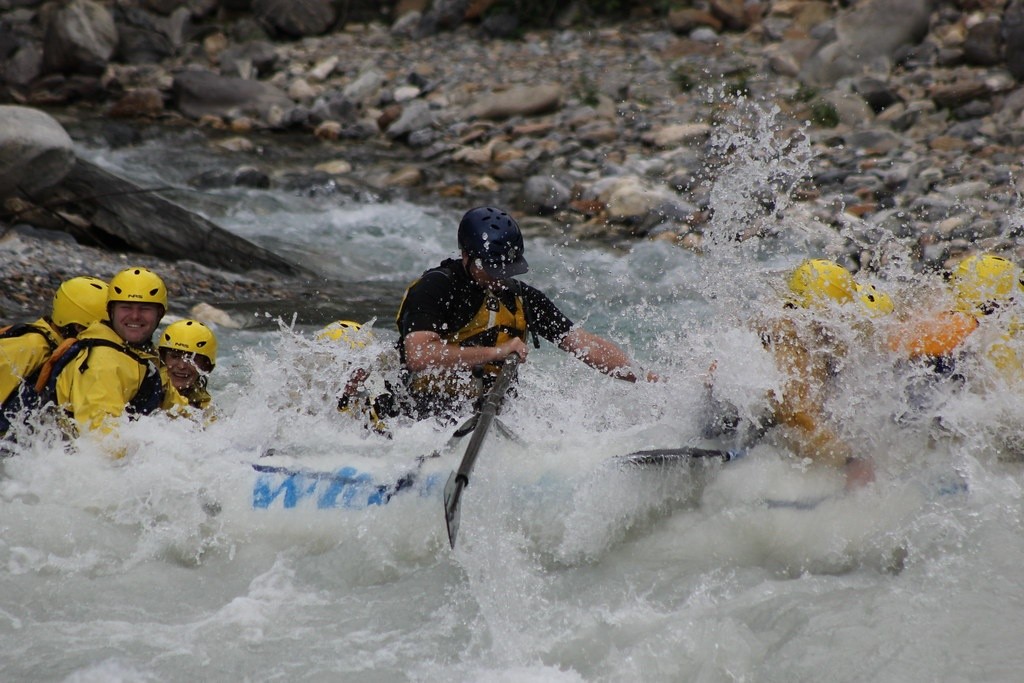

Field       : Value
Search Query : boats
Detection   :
[247,409,743,575]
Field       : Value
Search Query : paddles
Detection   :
[440,348,525,553]
[616,406,775,470]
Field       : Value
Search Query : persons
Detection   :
[0,267,218,461]
[369,206,668,426]
[315,321,388,435]
[704,254,1023,483]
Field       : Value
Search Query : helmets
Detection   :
[316,320,373,349]
[458,207,529,280]
[947,255,1024,318]
[785,259,857,309]
[51,275,109,328]
[158,320,217,372]
[106,267,168,320]
[855,282,894,318]
